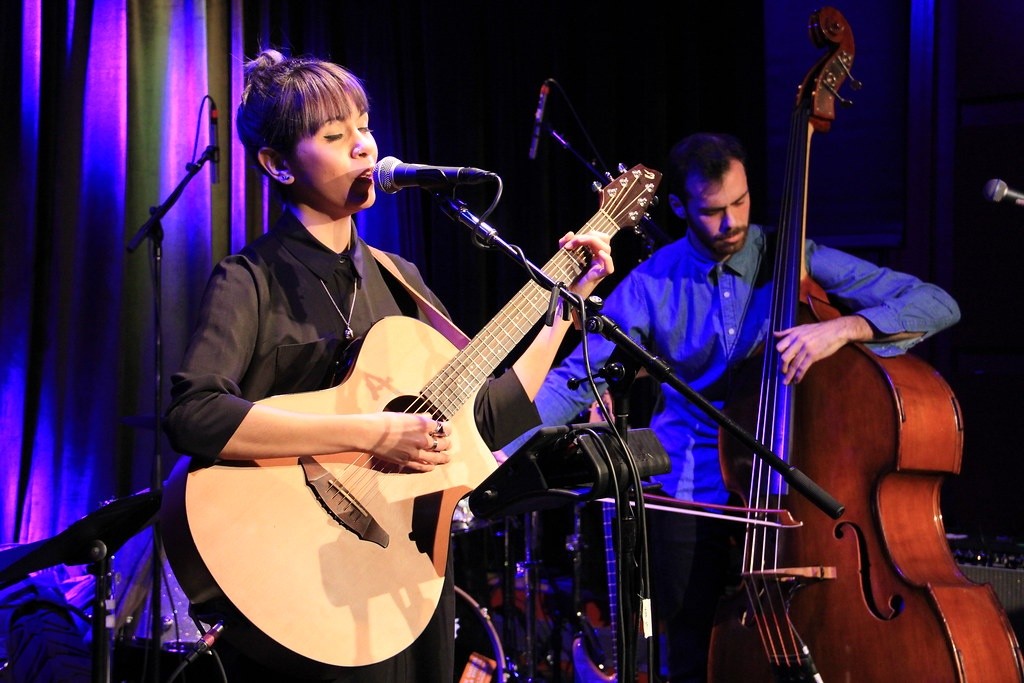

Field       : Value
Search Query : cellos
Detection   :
[705,5,1024,683]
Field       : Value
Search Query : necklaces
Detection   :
[319,278,357,340]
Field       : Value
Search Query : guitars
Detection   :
[159,161,664,670]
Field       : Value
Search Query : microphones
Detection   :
[372,156,497,195]
[210,102,220,184]
[982,178,1024,203]
[528,81,550,158]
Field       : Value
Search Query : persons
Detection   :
[495,133,961,682]
[160,49,614,683]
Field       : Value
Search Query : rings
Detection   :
[437,421,445,436]
[430,437,438,449]
